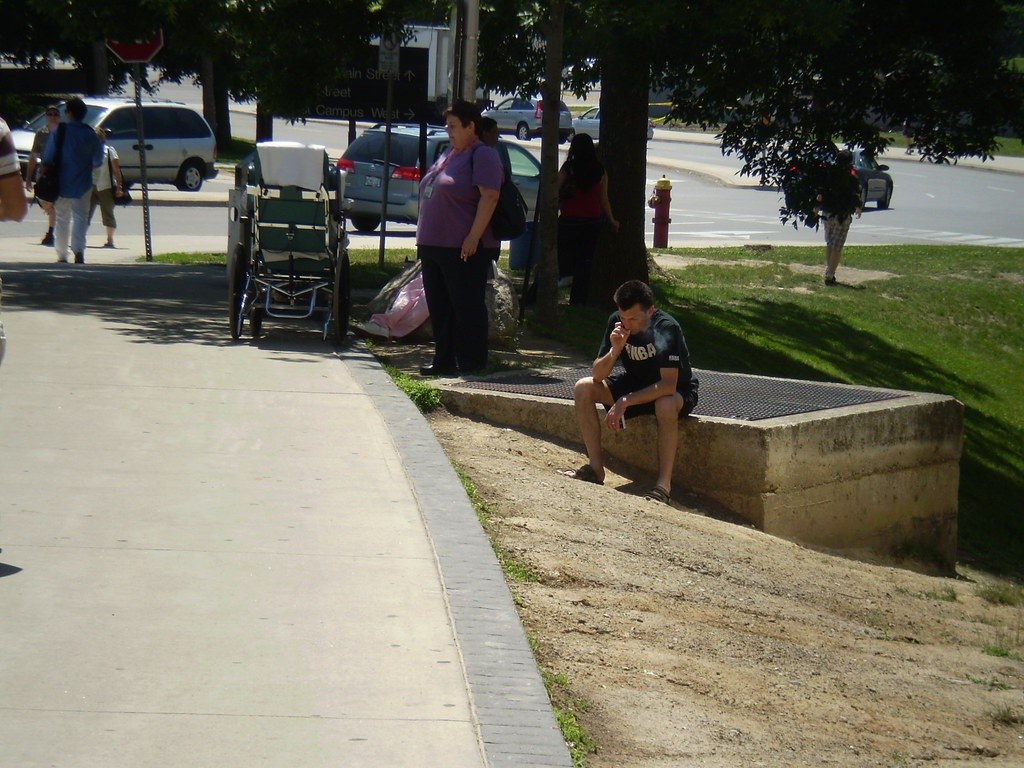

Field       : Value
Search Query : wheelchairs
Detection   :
[230,140,352,341]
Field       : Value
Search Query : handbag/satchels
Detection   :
[111,185,133,205]
[34,167,59,202]
[472,147,528,240]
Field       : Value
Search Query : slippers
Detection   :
[569,465,603,485]
[646,487,670,504]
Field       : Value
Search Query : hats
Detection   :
[45,105,60,115]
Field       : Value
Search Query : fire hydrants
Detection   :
[648,175,672,248]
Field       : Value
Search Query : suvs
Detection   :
[481,97,574,144]
[11,97,218,192]
[337,123,542,232]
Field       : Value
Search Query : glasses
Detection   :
[46,113,61,117]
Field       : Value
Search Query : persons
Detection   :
[814,151,862,283]
[26,106,61,244]
[564,279,700,505]
[44,98,103,264]
[476,116,500,147]
[88,127,123,248]
[416,100,501,376]
[558,133,620,306]
[0,118,27,362]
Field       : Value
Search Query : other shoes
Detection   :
[455,362,487,375]
[420,364,459,376]
[42,231,53,244]
[76,251,84,263]
[106,241,115,247]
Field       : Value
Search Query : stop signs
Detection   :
[105,27,164,64]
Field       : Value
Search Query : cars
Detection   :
[567,107,653,143]
[782,148,893,209]
